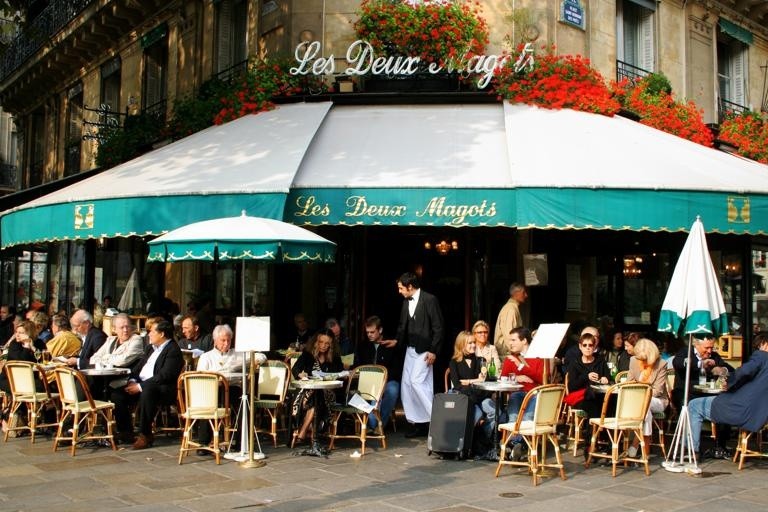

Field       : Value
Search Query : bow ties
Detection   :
[408,297,413,300]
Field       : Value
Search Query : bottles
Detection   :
[696,359,706,388]
[710,377,727,389]
[487,358,496,382]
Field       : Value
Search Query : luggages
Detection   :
[427,389,474,460]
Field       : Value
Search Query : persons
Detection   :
[382,272,448,440]
[448,282,766,471]
[1,293,404,460]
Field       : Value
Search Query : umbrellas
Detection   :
[657,215,733,469]
[117,268,144,313]
[145,210,337,454]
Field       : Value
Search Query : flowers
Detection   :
[165,46,328,145]
[348,1,490,84]
[490,32,768,165]
[95,112,165,170]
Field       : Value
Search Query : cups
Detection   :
[34,349,50,364]
[508,372,516,384]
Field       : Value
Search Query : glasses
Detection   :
[583,344,593,347]
[478,331,488,333]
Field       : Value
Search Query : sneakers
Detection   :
[628,446,639,457]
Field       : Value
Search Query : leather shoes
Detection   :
[134,437,153,449]
[669,451,700,463]
